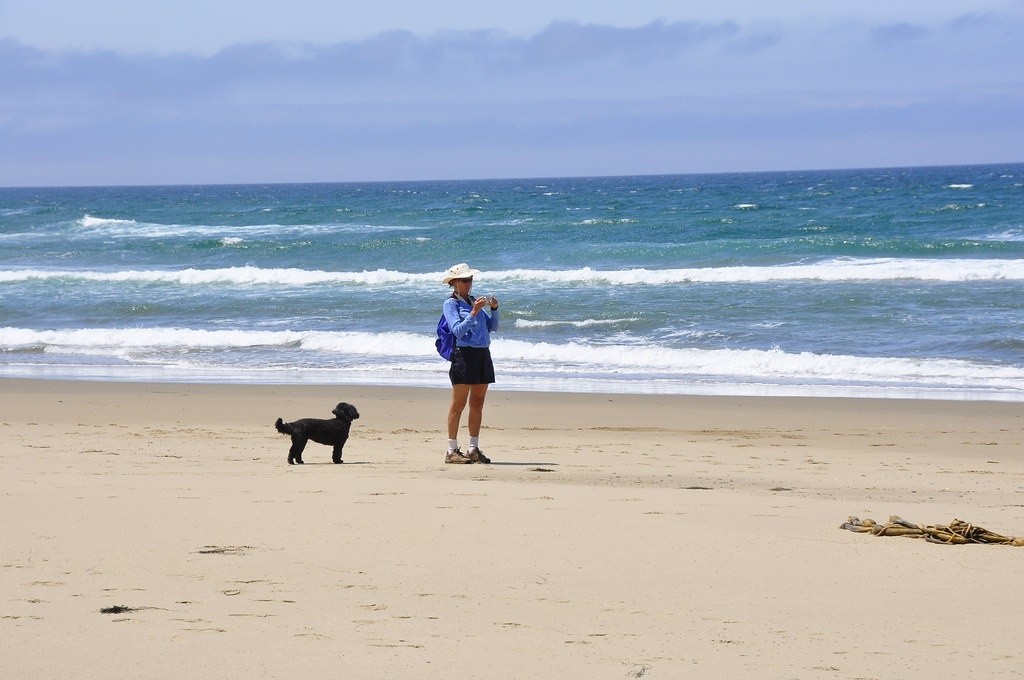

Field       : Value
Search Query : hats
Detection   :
[442,263,473,284]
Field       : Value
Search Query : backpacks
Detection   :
[435,297,490,361]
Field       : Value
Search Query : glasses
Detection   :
[459,278,473,283]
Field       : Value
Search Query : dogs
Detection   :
[275,402,359,464]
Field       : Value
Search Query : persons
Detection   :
[443,263,499,464]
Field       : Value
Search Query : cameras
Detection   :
[483,296,492,303]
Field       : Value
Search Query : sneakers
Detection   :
[445,447,471,464]
[466,447,490,463]
